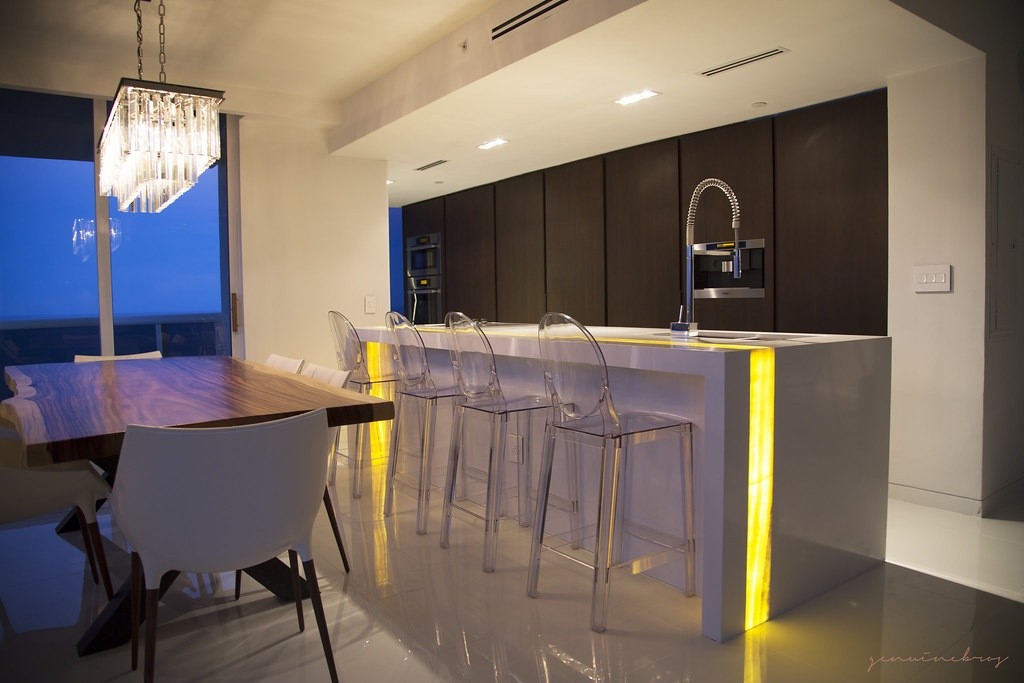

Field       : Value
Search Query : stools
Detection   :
[323,309,405,501]
[437,311,582,580]
[524,311,701,638]
[378,312,509,544]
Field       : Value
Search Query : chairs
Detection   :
[74,351,163,363]
[109,402,343,683]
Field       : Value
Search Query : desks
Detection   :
[347,319,894,647]
[0,354,396,657]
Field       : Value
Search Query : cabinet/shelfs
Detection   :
[544,139,679,328]
[682,87,887,335]
[444,171,544,323]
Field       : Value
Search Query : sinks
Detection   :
[652,328,822,343]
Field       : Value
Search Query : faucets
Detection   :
[670,178,741,329]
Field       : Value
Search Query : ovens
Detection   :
[406,275,442,327]
[406,233,441,276]
[689,238,768,297]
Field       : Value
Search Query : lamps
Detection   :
[93,76,227,216]
[71,216,122,264]
